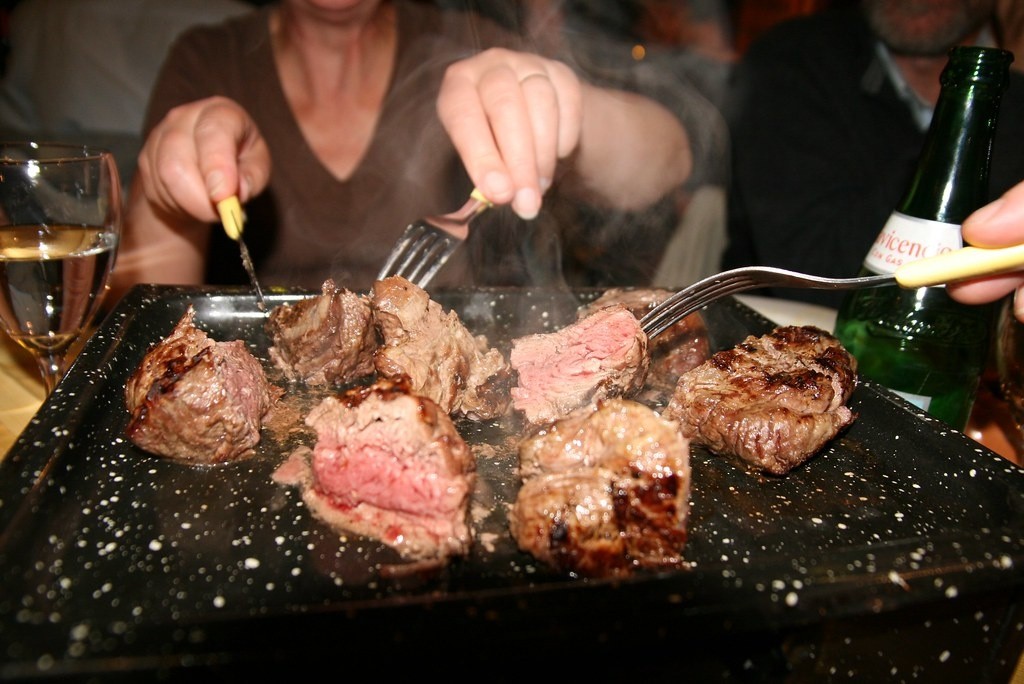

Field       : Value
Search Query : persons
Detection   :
[105,0,708,316]
[725,0,1023,315]
[942,179,1024,326]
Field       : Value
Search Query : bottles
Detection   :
[833,46,1024,431]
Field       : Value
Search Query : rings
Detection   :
[519,73,550,84]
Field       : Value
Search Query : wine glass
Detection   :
[0,144,121,396]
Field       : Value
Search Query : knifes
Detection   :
[218,200,273,316]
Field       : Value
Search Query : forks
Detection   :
[638,245,1023,340]
[370,186,496,286]
